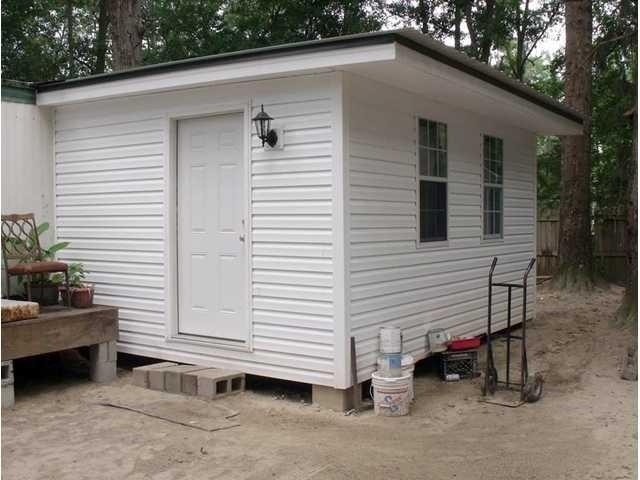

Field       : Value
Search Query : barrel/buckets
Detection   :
[369,371,411,416]
[377,354,416,399]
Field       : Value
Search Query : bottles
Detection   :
[441,373,460,382]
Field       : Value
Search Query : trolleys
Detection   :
[481,257,543,404]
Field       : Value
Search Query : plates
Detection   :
[443,337,481,350]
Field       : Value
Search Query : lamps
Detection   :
[251,104,278,148]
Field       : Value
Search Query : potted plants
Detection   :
[52,262,94,309]
